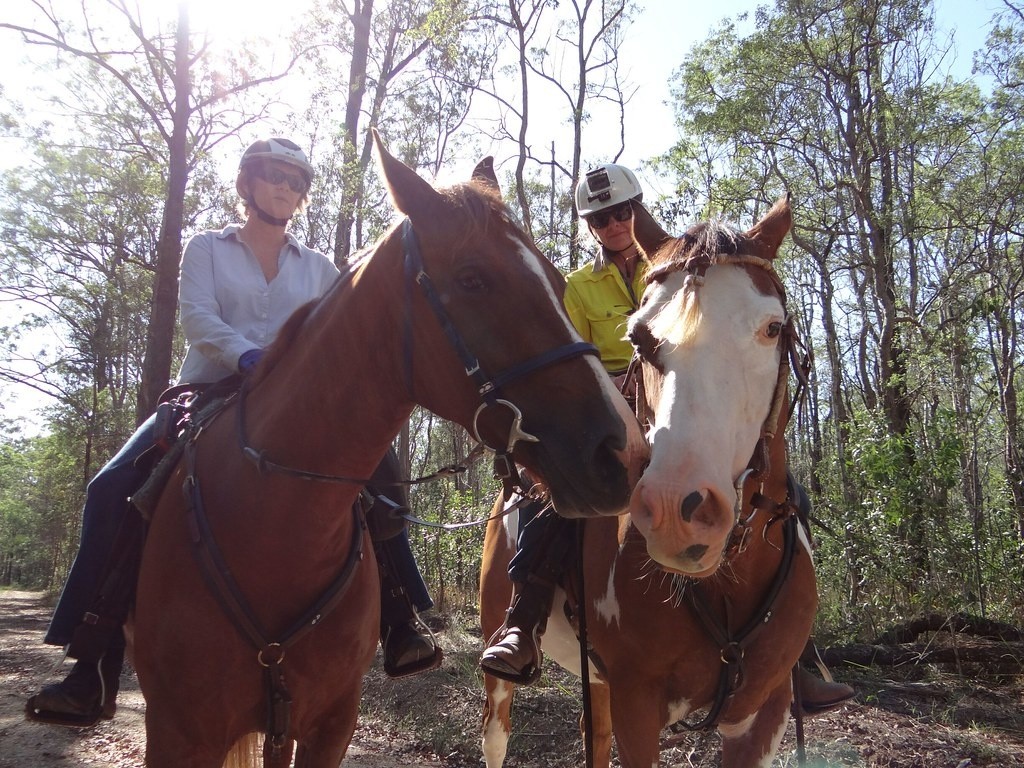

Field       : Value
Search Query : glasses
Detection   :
[588,202,632,230]
[251,165,305,194]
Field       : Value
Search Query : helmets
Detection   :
[238,138,313,182]
[574,163,643,216]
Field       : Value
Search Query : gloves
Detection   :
[239,349,267,377]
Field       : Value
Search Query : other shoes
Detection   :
[481,627,537,677]
[26,644,125,725]
[378,589,435,672]
[793,662,856,712]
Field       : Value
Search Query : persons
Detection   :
[26,140,435,722]
[481,164,856,711]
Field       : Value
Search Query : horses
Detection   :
[481,190,818,768]
[133,126,656,768]
